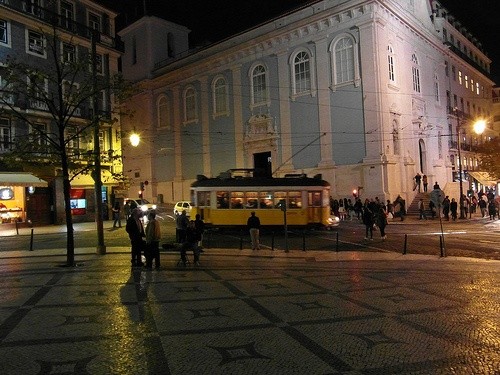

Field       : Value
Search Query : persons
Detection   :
[177,210,189,228]
[434,182,439,189]
[462,188,496,220]
[194,213,204,242]
[399,199,404,221]
[413,173,421,192]
[329,195,394,240]
[419,203,427,220]
[450,198,457,220]
[422,175,427,192]
[124,201,161,270]
[442,196,450,221]
[429,201,436,217]
[247,211,261,250]
[111,199,122,228]
[219,198,296,208]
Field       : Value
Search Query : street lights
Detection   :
[457,120,487,220]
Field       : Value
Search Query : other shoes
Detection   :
[133,262,137,266]
[138,262,144,265]
[144,265,152,268]
[155,266,160,269]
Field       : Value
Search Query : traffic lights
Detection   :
[140,182,144,191]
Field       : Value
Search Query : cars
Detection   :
[174,201,193,216]
[329,214,340,228]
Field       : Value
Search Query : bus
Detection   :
[189,132,331,237]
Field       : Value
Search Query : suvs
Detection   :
[123,198,157,214]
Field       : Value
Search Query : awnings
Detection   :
[67,168,119,189]
[468,172,500,187]
[0,173,48,187]
